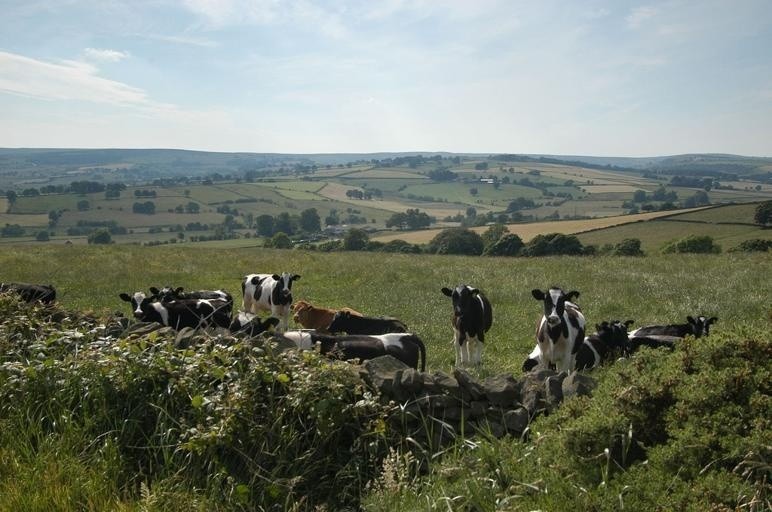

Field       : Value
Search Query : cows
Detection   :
[0,280,58,307]
[119,284,232,343]
[532,289,587,376]
[521,318,635,374]
[440,283,493,369]
[622,313,719,372]
[278,301,427,375]
[239,270,302,334]
[209,307,281,341]
